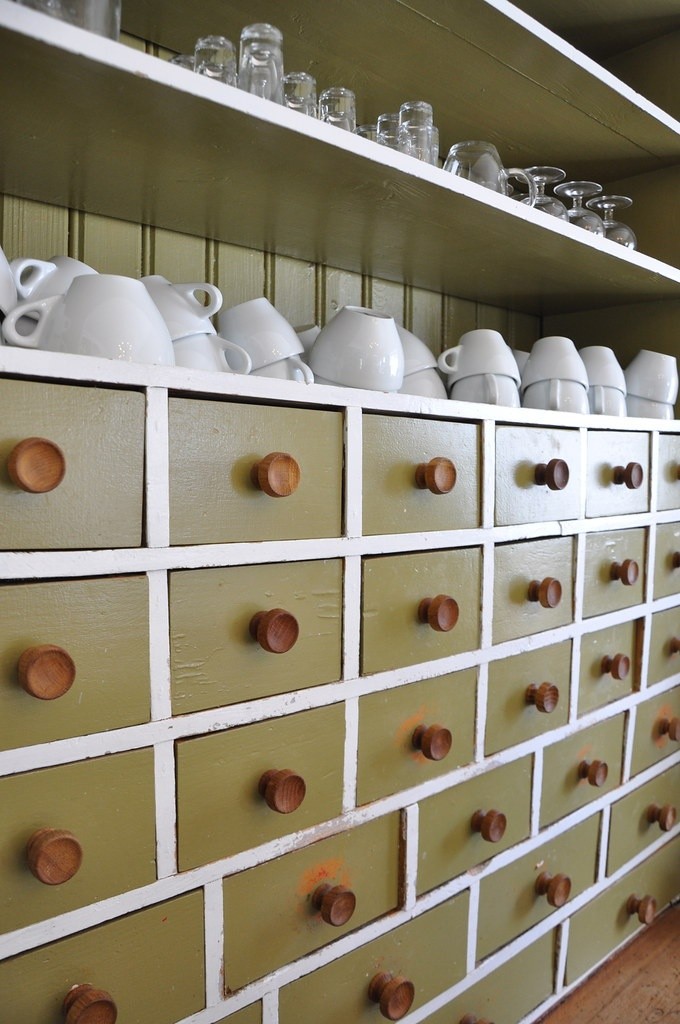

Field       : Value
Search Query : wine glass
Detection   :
[586,196,638,250]
[517,165,572,221]
[554,182,607,238]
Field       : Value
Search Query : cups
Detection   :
[0,247,405,399]
[422,126,439,167]
[237,22,285,105]
[378,114,400,150]
[194,36,236,87]
[353,126,377,142]
[400,101,432,164]
[283,72,317,117]
[403,369,450,400]
[318,87,355,134]
[170,54,194,71]
[394,321,439,376]
[441,141,536,206]
[436,329,680,421]
[15,0,121,44]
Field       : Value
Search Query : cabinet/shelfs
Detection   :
[0,0,680,1024]
